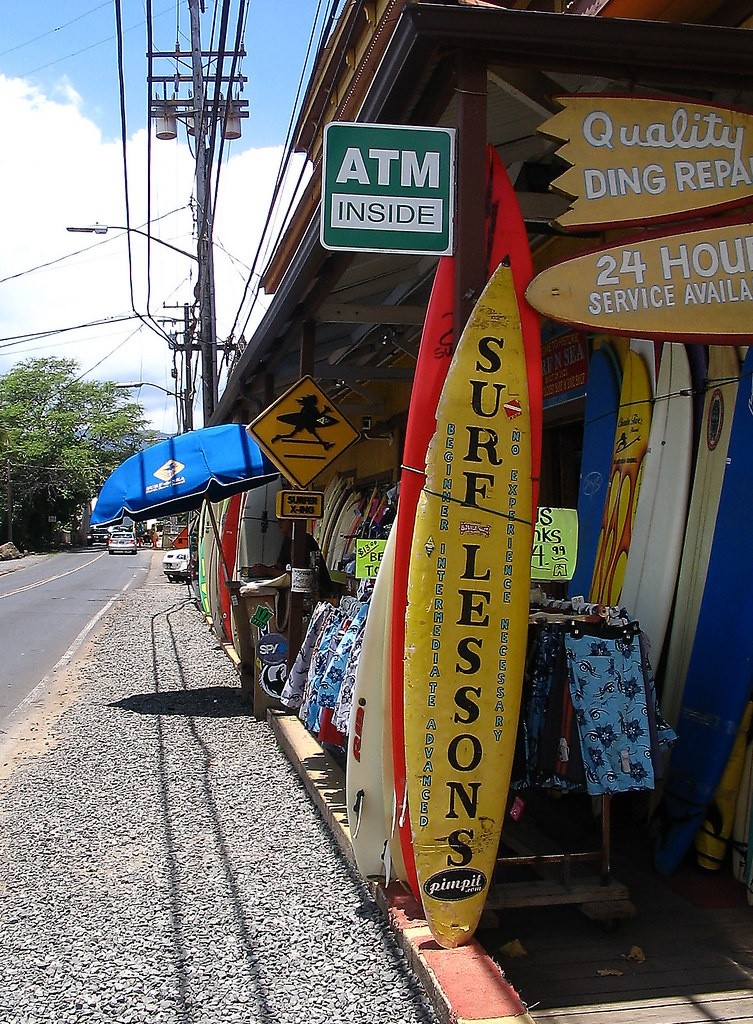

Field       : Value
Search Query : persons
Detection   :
[251,519,332,585]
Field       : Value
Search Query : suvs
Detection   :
[108,531,138,555]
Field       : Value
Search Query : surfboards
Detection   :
[349,254,455,905]
[197,476,284,636]
[401,253,532,948]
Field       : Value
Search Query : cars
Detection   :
[162,547,192,583]
[86,529,109,547]
[143,535,152,545]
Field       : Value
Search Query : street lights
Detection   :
[67,223,214,429]
[114,383,193,434]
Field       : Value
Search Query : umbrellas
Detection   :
[89,423,280,583]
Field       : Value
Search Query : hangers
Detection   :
[528,598,628,626]
